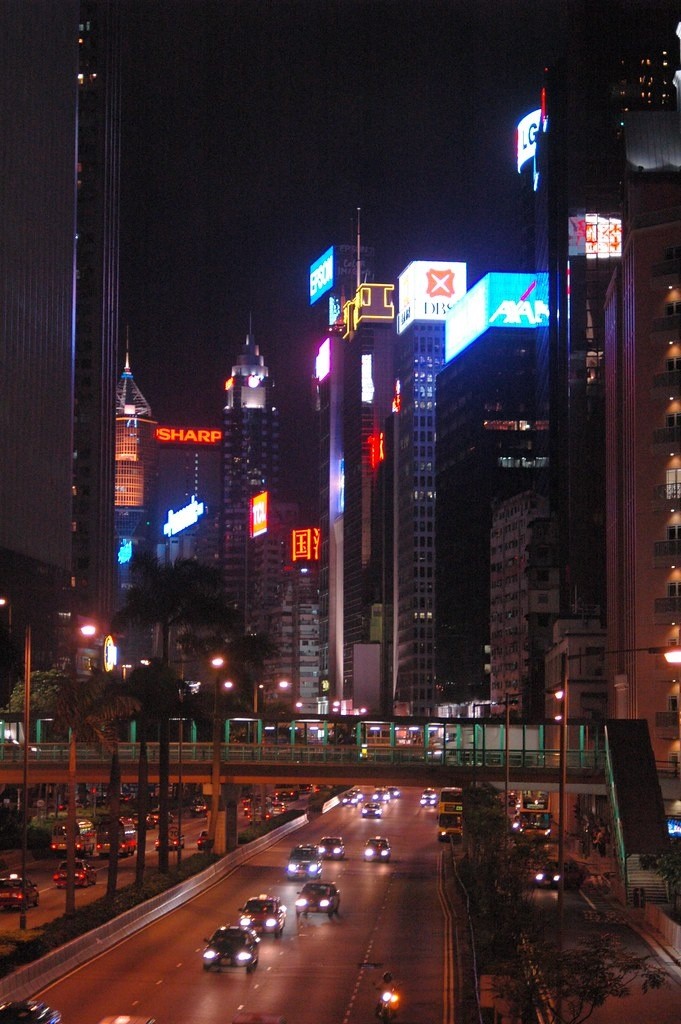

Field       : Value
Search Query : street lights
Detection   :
[210,652,231,843]
[541,642,680,961]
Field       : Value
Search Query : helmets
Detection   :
[382,971,393,983]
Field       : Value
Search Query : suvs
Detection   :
[285,843,323,880]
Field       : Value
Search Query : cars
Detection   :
[359,803,383,818]
[317,834,344,859]
[157,831,184,850]
[202,922,259,973]
[239,895,288,939]
[0,873,40,912]
[361,834,392,861]
[97,1014,158,1024]
[0,999,64,1024]
[128,813,154,829]
[536,860,582,890]
[240,783,313,824]
[373,789,390,804]
[293,881,340,921]
[342,792,357,807]
[353,789,364,801]
[190,801,207,816]
[198,830,212,847]
[55,858,99,887]
[419,787,439,806]
[388,786,401,798]
[149,807,174,825]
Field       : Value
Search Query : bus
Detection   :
[514,789,553,847]
[52,819,97,854]
[91,816,137,858]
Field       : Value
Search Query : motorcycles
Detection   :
[373,983,400,1022]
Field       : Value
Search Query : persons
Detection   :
[587,816,611,857]
[376,972,398,1016]
